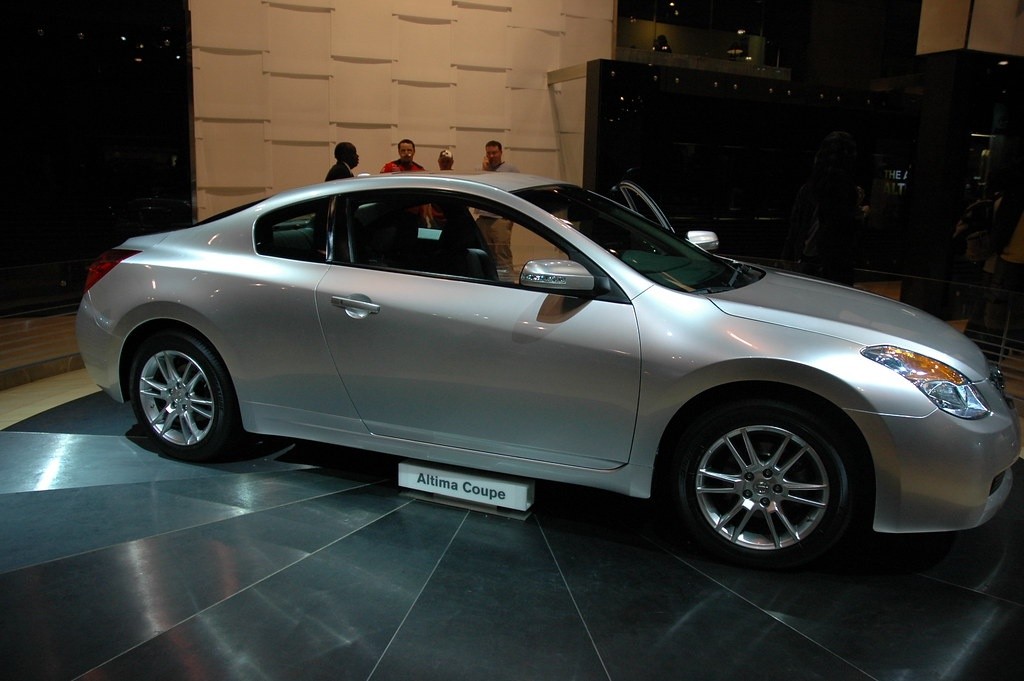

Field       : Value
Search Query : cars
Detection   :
[76,171,1021,571]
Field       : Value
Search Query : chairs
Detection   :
[435,209,500,281]
[362,200,428,272]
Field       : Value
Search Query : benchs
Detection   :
[272,227,315,251]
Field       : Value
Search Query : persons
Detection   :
[315,142,360,262]
[380,139,433,223]
[783,130,870,284]
[439,149,454,170]
[652,35,672,52]
[475,141,519,271]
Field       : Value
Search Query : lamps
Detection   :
[727,41,743,55]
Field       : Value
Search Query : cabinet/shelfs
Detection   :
[581,47,1024,277]
